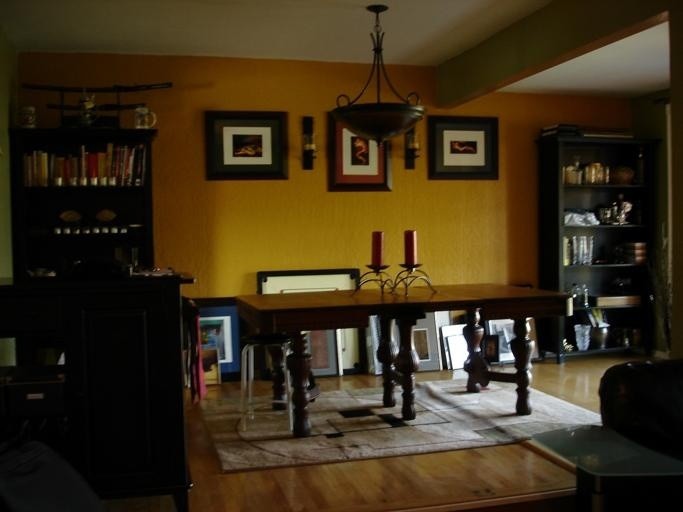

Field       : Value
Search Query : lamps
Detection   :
[403,111,421,170]
[300,115,317,170]
[329,1,426,141]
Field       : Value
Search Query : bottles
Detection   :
[566,282,588,308]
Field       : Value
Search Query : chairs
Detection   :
[564,357,683,512]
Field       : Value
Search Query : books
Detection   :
[563,161,611,185]
[17,143,148,235]
[563,235,595,266]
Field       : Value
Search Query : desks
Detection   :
[234,280,566,434]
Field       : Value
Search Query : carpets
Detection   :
[199,375,604,473]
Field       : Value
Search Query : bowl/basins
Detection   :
[590,327,608,350]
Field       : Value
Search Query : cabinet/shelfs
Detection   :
[0,273,200,507]
[538,124,654,360]
[10,124,159,275]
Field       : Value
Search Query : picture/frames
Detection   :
[203,108,291,181]
[325,110,392,192]
[426,115,501,182]
[254,269,367,379]
[369,311,444,374]
[301,326,344,377]
[180,297,245,382]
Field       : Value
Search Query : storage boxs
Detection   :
[586,295,641,307]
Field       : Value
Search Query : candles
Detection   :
[370,228,383,267]
[403,229,417,264]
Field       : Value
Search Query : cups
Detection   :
[574,324,590,351]
[133,105,156,129]
[21,106,35,128]
